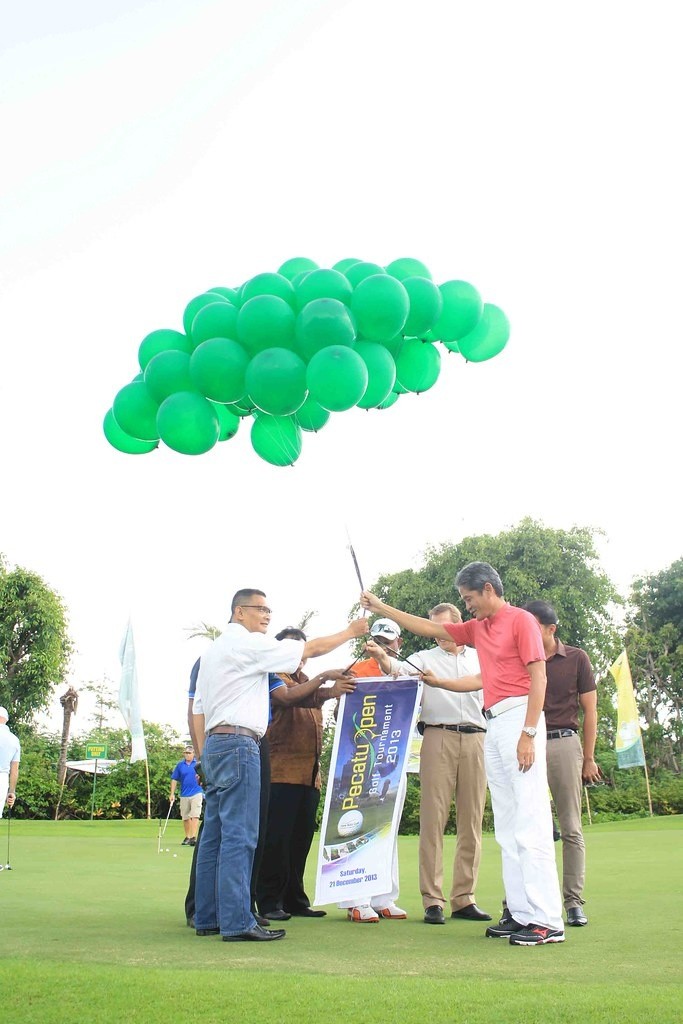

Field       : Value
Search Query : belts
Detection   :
[208,726,258,743]
[425,724,486,733]
[547,729,572,739]
[482,695,529,720]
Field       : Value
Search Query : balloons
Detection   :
[103,256,509,467]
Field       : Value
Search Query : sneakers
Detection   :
[500,910,513,925]
[485,916,523,937]
[510,922,565,947]
[347,906,380,924]
[377,905,407,919]
[568,906,587,926]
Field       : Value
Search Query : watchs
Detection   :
[522,727,536,737]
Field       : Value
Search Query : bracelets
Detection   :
[317,674,326,684]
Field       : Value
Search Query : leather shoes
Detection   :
[258,916,271,924]
[263,910,291,921]
[424,905,445,924]
[450,904,491,921]
[224,924,285,941]
[292,907,326,917]
[196,928,219,935]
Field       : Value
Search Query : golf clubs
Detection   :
[5,795,13,871]
[158,801,174,838]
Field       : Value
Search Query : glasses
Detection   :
[241,605,272,615]
[369,624,399,637]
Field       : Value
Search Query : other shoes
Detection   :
[190,837,196,846]
[182,837,189,845]
[186,916,194,924]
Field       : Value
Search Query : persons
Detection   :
[360,562,564,945]
[256,628,356,919]
[499,601,602,925]
[170,745,204,846]
[334,617,407,922]
[185,622,359,928]
[0,707,20,871]
[363,602,492,923]
[191,589,369,941]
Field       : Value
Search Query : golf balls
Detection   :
[337,810,364,837]
[160,849,163,852]
[166,849,169,852]
[173,853,177,857]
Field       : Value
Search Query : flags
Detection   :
[118,625,147,762]
[610,651,645,768]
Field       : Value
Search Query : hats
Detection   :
[0,707,9,721]
[183,746,194,753]
[370,619,401,640]
